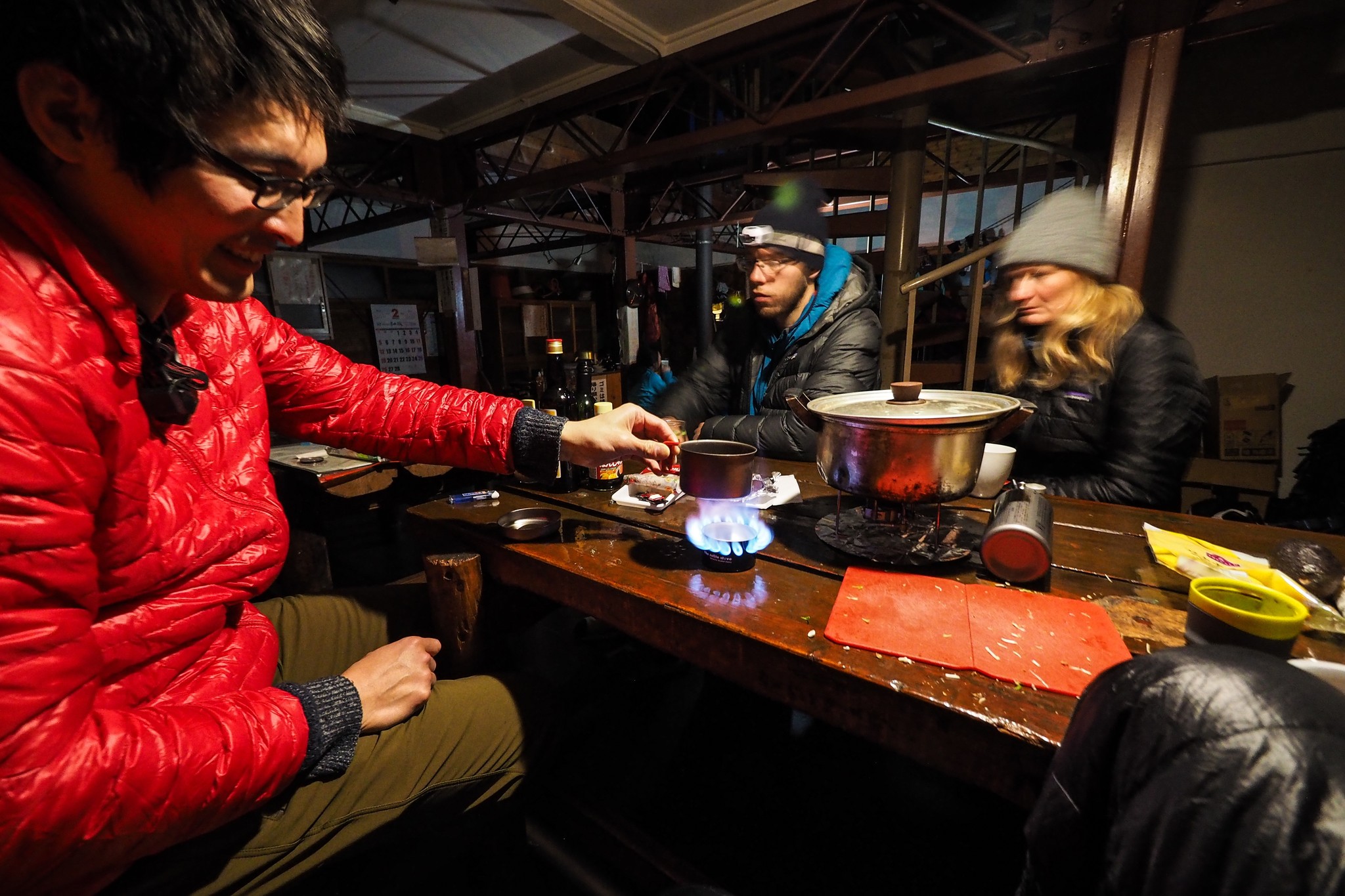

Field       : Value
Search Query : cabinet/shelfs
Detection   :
[497,297,598,407]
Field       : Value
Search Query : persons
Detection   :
[1028,642,1345,895]
[982,185,1208,513]
[637,194,882,482]
[0,0,686,896]
[630,351,675,414]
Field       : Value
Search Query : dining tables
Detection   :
[267,434,1345,821]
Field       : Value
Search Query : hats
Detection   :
[737,179,826,271]
[996,186,1118,283]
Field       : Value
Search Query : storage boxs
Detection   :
[1200,368,1295,466]
[1180,456,1277,523]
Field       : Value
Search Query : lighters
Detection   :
[448,490,499,504]
[635,491,668,504]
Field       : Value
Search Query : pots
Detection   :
[803,381,1020,505]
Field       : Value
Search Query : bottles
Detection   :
[590,403,624,492]
[570,347,594,486]
[542,333,578,495]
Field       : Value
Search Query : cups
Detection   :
[1182,576,1310,663]
[660,438,757,501]
[971,443,1017,499]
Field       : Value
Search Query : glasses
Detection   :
[97,92,337,210]
[734,252,819,274]
